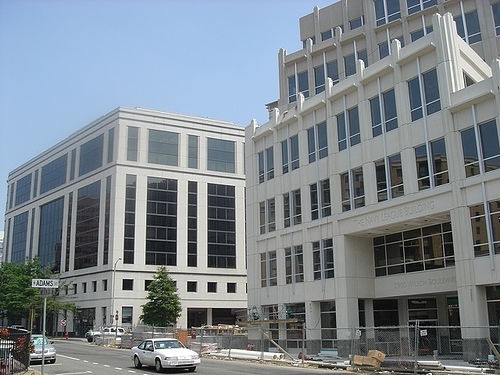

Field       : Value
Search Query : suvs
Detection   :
[84,327,132,342]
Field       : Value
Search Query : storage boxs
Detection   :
[353,350,386,368]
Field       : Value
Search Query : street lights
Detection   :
[111,257,123,326]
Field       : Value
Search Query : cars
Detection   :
[10,324,25,330]
[0,334,57,368]
[130,338,201,373]
